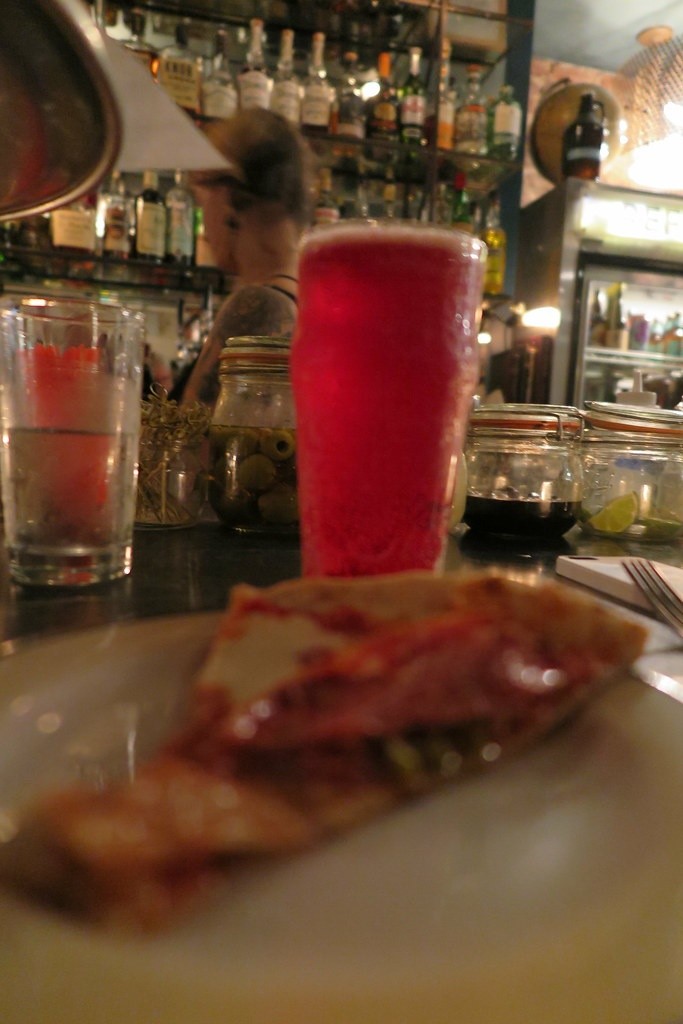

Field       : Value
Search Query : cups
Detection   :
[293,223,488,577]
[136,426,205,529]
[0,294,147,588]
[207,337,294,535]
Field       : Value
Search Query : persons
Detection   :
[148,104,313,404]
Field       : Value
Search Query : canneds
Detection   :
[628,318,648,352]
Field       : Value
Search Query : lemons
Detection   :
[585,491,640,534]
[642,517,681,537]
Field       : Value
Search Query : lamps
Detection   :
[521,257,562,405]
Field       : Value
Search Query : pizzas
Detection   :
[24,565,653,935]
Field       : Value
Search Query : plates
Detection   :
[0,613,682,1022]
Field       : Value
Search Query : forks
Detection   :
[622,559,683,640]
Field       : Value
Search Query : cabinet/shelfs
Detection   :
[0,0,536,405]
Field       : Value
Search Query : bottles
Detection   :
[437,38,524,160]
[52,168,198,266]
[118,14,428,144]
[561,90,603,180]
[587,278,683,354]
[616,368,657,406]
[317,161,507,298]
[462,401,683,544]
